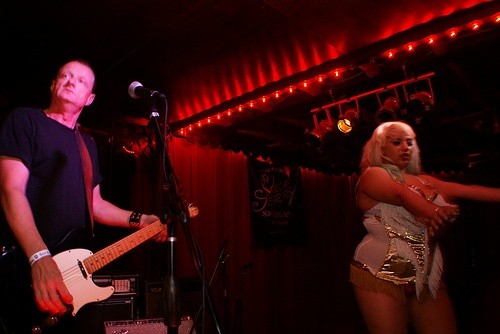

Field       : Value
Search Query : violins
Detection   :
[52,205,202,316]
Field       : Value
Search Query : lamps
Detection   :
[308,119,337,147]
[376,97,398,122]
[336,108,360,134]
[406,91,431,117]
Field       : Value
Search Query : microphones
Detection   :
[128,81,165,98]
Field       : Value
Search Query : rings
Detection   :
[435,206,440,213]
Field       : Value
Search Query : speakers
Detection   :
[73,276,206,334]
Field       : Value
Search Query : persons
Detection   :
[0,60,167,333]
[350,121,499,334]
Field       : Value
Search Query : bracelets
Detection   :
[29,249,52,267]
[129,211,145,230]
